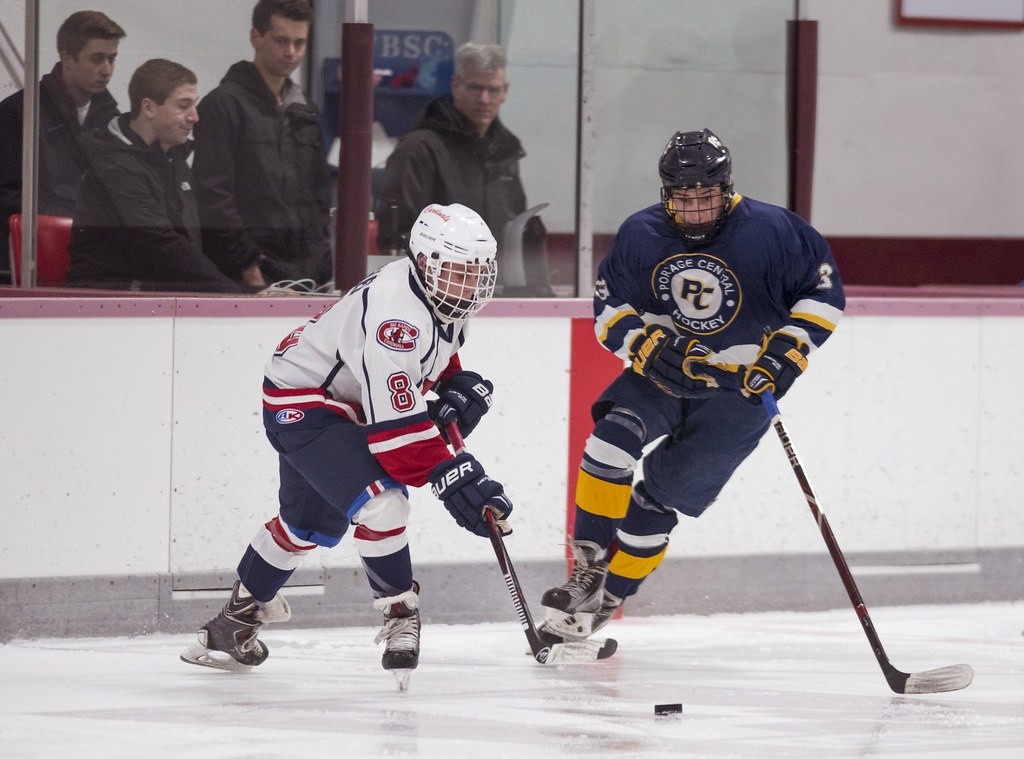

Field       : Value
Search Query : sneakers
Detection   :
[180,580,291,671]
[541,540,610,622]
[538,585,626,644]
[373,580,420,691]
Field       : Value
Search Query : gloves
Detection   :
[428,371,494,446]
[628,322,720,399]
[429,453,513,537]
[737,325,810,407]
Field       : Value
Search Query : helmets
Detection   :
[659,128,734,246]
[407,203,498,324]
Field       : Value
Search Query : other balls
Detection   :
[653,702,683,717]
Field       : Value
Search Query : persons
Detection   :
[542,128,845,639]
[0,11,126,288]
[65,59,245,293]
[374,40,527,298]
[192,0,331,292]
[197,202,512,669]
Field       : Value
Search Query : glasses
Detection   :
[459,78,505,96]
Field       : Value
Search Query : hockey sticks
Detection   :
[441,417,619,667]
[759,386,975,696]
